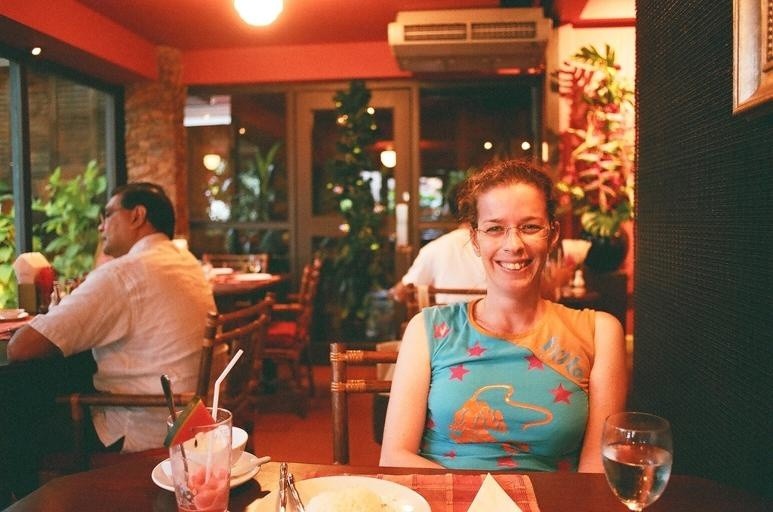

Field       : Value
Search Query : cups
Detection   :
[165,407,232,512]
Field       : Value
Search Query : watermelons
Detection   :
[163,395,216,448]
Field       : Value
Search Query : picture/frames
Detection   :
[727,1,772,117]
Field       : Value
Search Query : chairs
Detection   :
[240,256,323,418]
[325,338,403,466]
[51,288,276,471]
[405,282,486,321]
[200,249,268,312]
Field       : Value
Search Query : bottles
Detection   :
[36,266,66,314]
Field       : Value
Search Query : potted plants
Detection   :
[316,78,396,335]
[549,44,638,272]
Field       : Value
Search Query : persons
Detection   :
[0,181,230,505]
[388,181,488,306]
[375,157,630,477]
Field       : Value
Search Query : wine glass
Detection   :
[599,409,674,512]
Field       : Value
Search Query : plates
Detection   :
[0,309,30,322]
[236,273,272,282]
[148,451,261,493]
[213,267,233,276]
[247,476,430,511]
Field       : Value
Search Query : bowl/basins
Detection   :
[228,426,249,467]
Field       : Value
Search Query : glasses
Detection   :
[475,222,550,237]
[99,206,124,222]
